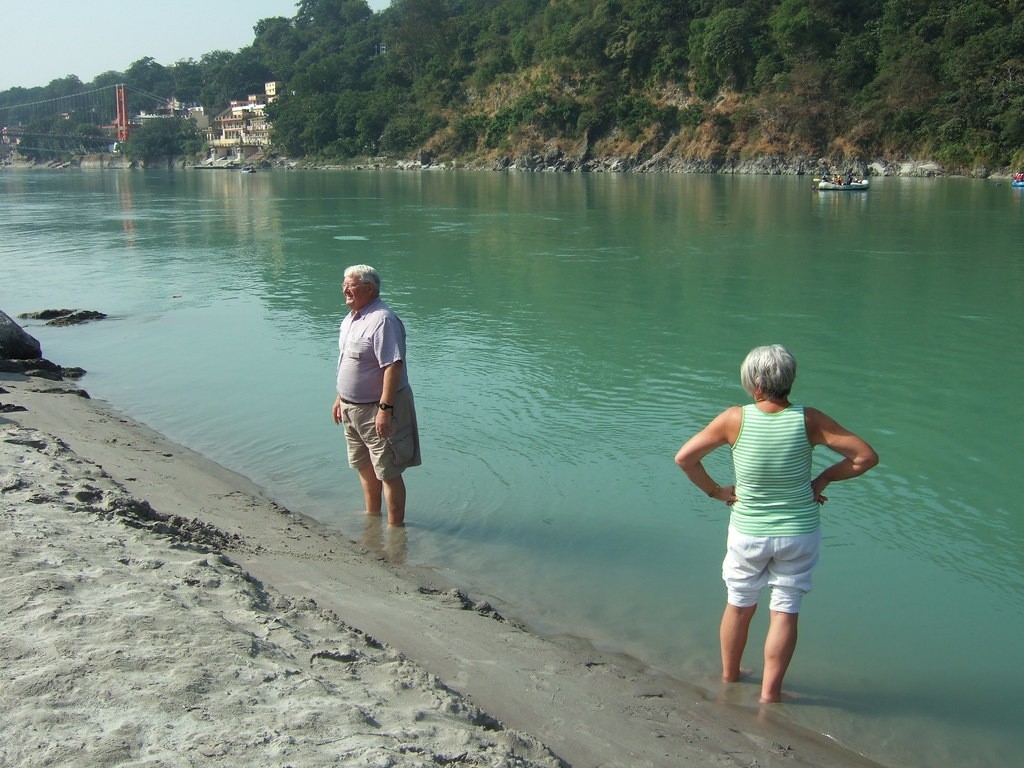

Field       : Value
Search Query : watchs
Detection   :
[708,484,720,498]
[378,403,394,411]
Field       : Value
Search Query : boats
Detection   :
[1012,181,1024,187]
[811,178,870,191]
[240,166,256,173]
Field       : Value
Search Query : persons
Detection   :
[332,265,422,531]
[822,174,852,186]
[1014,173,1024,182]
[675,344,880,706]
